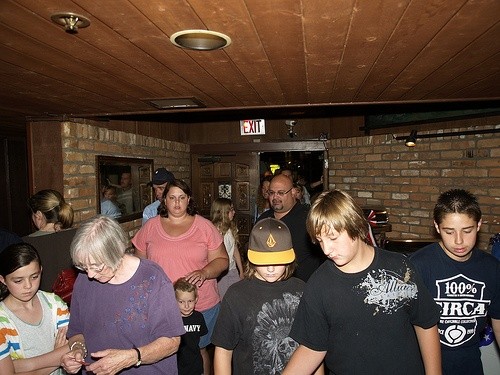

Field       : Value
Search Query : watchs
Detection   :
[132,348,141,367]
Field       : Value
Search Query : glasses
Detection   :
[73,261,105,272]
[266,187,293,197]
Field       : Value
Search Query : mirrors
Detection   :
[95,155,156,224]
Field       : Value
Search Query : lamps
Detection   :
[405,129,417,146]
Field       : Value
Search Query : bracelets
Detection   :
[70,341,87,358]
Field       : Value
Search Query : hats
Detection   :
[147,168,174,186]
[248,217,296,265]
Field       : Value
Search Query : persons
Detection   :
[281,190,442,375]
[255,169,326,284]
[142,168,174,225]
[100,169,142,219]
[209,218,324,375]
[407,190,500,375]
[210,198,245,300]
[0,242,70,375]
[23,189,74,238]
[172,276,208,375]
[131,180,229,375]
[66,215,187,375]
